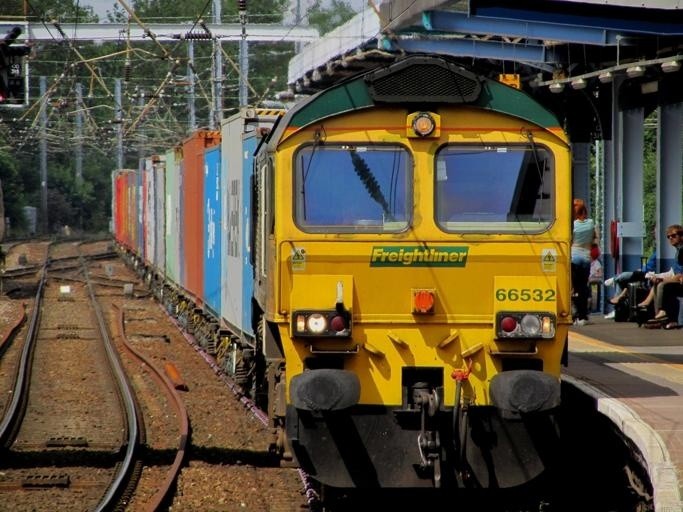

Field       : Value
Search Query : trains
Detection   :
[112,56,571,488]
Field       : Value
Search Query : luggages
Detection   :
[630,256,654,327]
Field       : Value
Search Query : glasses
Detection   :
[667,234,678,239]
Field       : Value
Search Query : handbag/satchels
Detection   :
[591,243,601,260]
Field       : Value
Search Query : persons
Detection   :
[571,198,597,327]
[602,224,683,330]
[588,242,603,284]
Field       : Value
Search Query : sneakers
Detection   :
[572,318,593,326]
[603,277,621,319]
[631,304,678,329]
